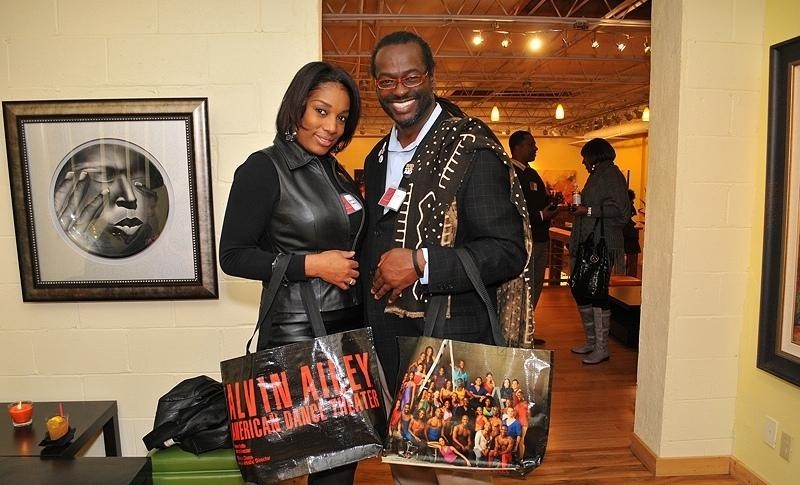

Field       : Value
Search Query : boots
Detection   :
[582,306,610,365]
[570,304,598,355]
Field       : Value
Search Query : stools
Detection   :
[607,285,642,351]
[147,443,246,484]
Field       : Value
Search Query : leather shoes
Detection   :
[533,338,545,345]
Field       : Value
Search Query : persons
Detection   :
[219,61,368,485]
[383,347,532,469]
[506,129,561,345]
[567,137,631,365]
[361,30,528,485]
[53,144,158,259]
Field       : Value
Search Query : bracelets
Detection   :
[412,249,423,278]
[587,207,592,217]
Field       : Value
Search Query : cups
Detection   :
[550,190,558,210]
[46,414,69,442]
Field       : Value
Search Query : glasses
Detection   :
[375,68,429,91]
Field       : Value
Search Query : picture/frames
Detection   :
[757,36,800,388]
[0,98,222,302]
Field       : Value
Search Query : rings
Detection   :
[349,280,354,284]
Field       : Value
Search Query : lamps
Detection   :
[464,26,653,53]
[487,93,651,137]
[360,116,388,136]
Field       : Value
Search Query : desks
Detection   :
[0,455,154,485]
[0,399,123,461]
[547,218,645,285]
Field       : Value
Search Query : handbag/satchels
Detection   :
[568,204,611,307]
[380,245,556,480]
[142,374,234,457]
[218,250,395,484]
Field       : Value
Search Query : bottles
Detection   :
[571,184,582,209]
